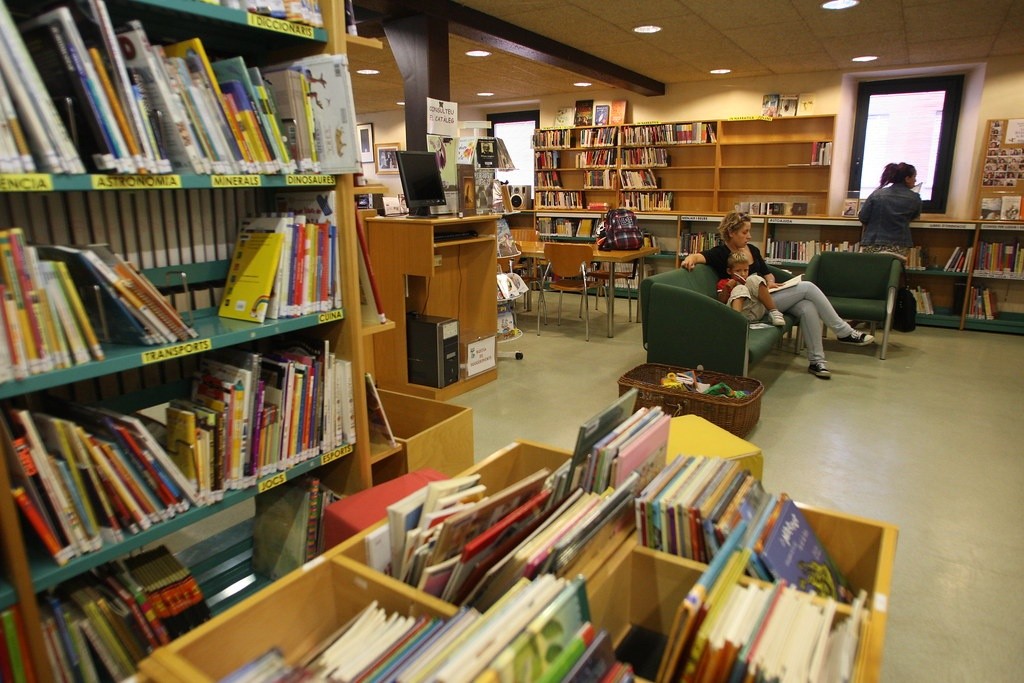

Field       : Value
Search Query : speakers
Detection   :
[510,185,532,211]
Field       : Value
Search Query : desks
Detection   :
[514,241,659,338]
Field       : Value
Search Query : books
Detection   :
[215,392,867,683]
[768,274,805,293]
[767,238,865,262]
[905,242,1024,320]
[981,196,1024,221]
[0,189,357,683]
[537,259,598,282]
[474,137,528,340]
[536,122,717,211]
[762,92,815,118]
[1,4,365,180]
[788,142,832,166]
[735,200,808,215]
[598,234,660,289]
[681,229,725,255]
[727,272,757,296]
[536,218,601,237]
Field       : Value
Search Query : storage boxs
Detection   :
[373,387,474,480]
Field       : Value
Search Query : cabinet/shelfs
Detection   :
[492,210,1024,334]
[534,113,838,216]
[1,0,395,683]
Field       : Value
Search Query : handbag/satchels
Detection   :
[893,287,917,332]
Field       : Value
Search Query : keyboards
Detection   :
[434,232,473,242]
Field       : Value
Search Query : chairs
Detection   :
[800,251,902,360]
[498,237,638,342]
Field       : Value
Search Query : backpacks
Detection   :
[600,209,643,250]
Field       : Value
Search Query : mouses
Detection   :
[466,230,478,237]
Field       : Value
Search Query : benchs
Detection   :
[641,263,802,378]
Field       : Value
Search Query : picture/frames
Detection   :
[374,142,402,174]
[356,122,374,163]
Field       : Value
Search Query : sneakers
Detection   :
[768,310,786,326]
[808,363,831,378]
[837,330,874,345]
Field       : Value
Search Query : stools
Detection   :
[663,414,764,485]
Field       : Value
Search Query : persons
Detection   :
[681,212,875,377]
[847,162,922,327]
[380,151,394,168]
[984,121,1024,186]
[717,252,785,326]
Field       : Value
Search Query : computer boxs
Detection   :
[405,312,461,390]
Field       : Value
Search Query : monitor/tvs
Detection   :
[396,150,447,219]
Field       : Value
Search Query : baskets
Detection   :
[618,360,763,439]
